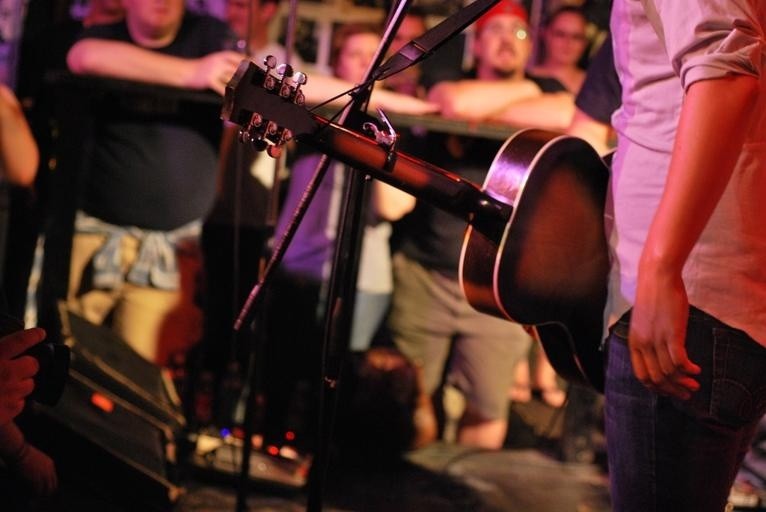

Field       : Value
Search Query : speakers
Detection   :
[50,301,185,427]
[29,362,176,491]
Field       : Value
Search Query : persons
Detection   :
[598,0,766,512]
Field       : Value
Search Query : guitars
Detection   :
[218,54,616,396]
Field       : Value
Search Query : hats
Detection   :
[475,1,529,34]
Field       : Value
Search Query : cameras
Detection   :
[0,311,72,407]
